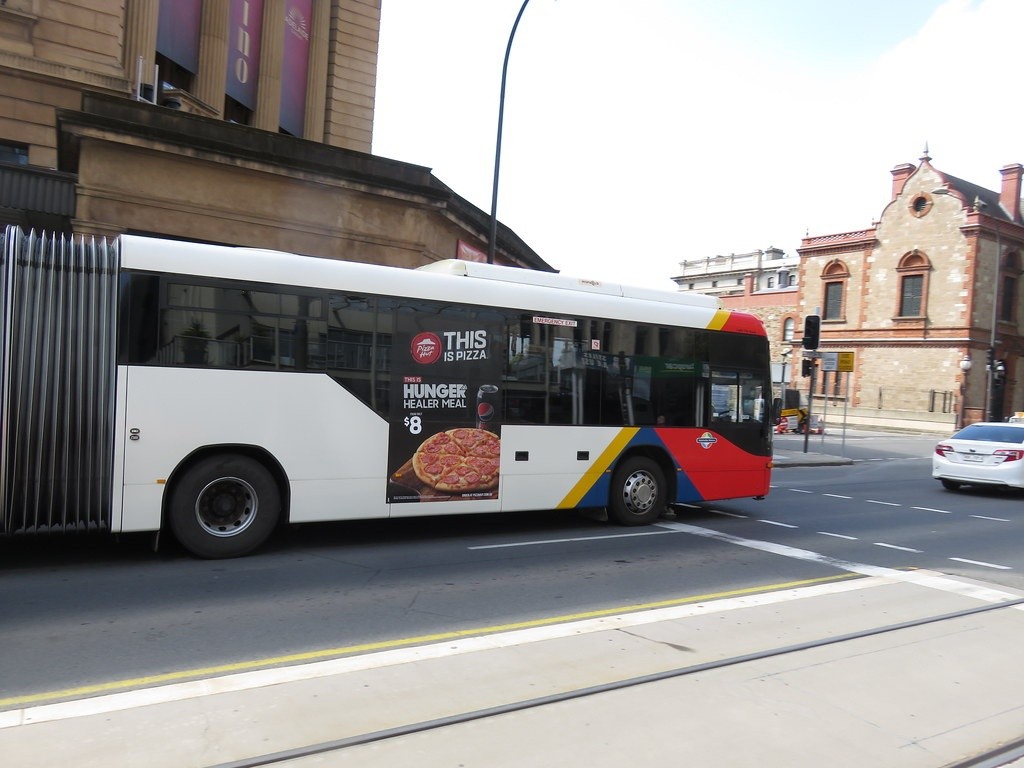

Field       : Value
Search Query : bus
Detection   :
[0,225,775,563]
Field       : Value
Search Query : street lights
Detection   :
[959,354,973,433]
[780,347,792,410]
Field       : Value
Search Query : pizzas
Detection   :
[412,428,501,494]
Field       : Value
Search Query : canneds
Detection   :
[475,384,499,424]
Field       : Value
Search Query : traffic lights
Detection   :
[802,359,811,378]
[985,346,994,365]
[802,314,821,350]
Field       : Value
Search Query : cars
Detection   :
[932,421,1024,494]
